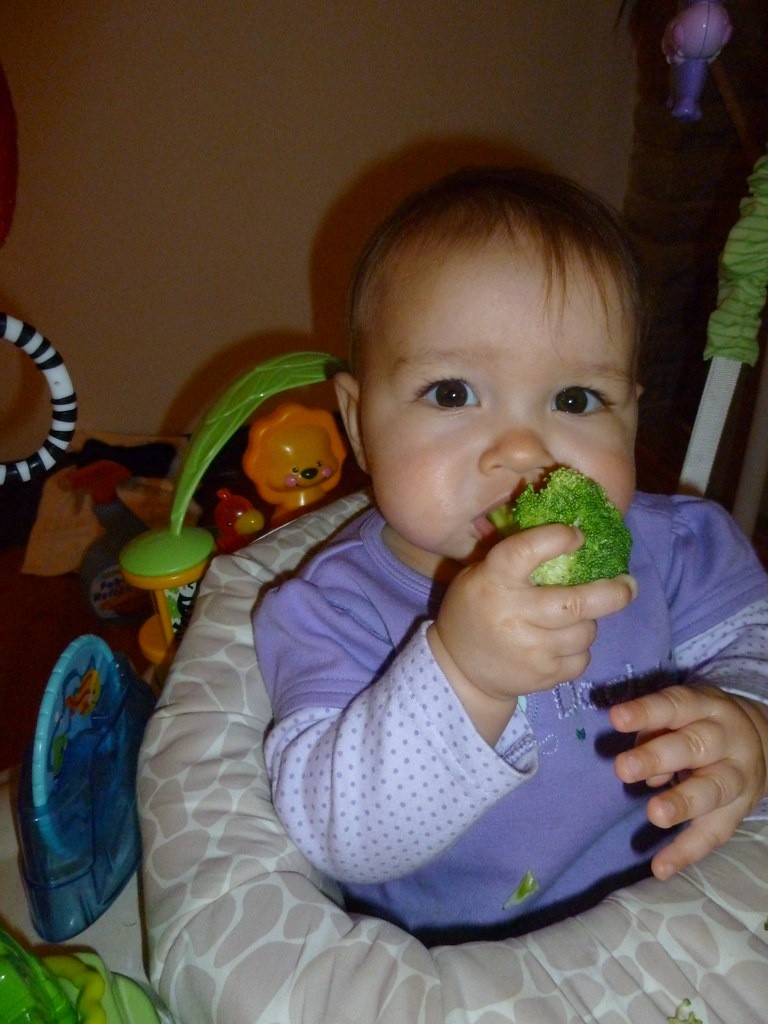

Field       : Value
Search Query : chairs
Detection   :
[136,486,768,1024]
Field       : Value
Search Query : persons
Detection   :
[252,168,768,947]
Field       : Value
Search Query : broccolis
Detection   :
[488,468,633,586]
